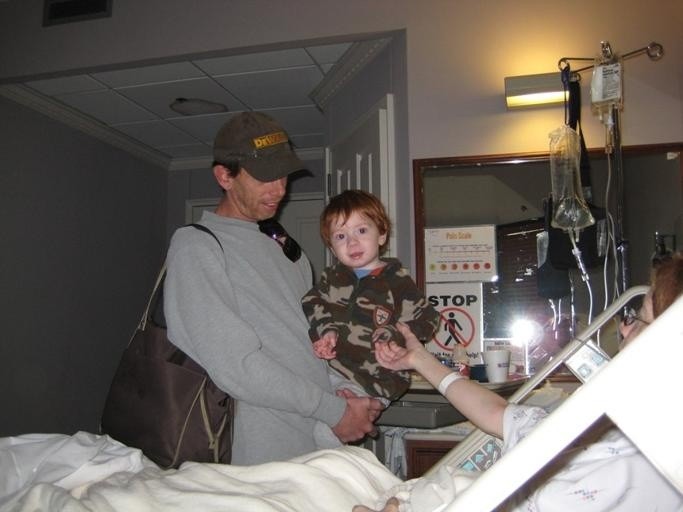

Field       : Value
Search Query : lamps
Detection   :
[504,73,570,110]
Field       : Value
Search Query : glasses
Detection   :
[622,306,651,328]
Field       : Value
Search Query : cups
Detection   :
[480,350,512,383]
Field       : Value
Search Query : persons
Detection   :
[352,252,683,512]
[161,112,383,468]
[301,191,438,448]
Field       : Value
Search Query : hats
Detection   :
[212,109,315,184]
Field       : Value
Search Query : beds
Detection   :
[0,283,683,510]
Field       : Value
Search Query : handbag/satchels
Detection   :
[98,223,234,474]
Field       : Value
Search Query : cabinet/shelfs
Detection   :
[398,437,472,480]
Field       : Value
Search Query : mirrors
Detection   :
[412,142,683,380]
